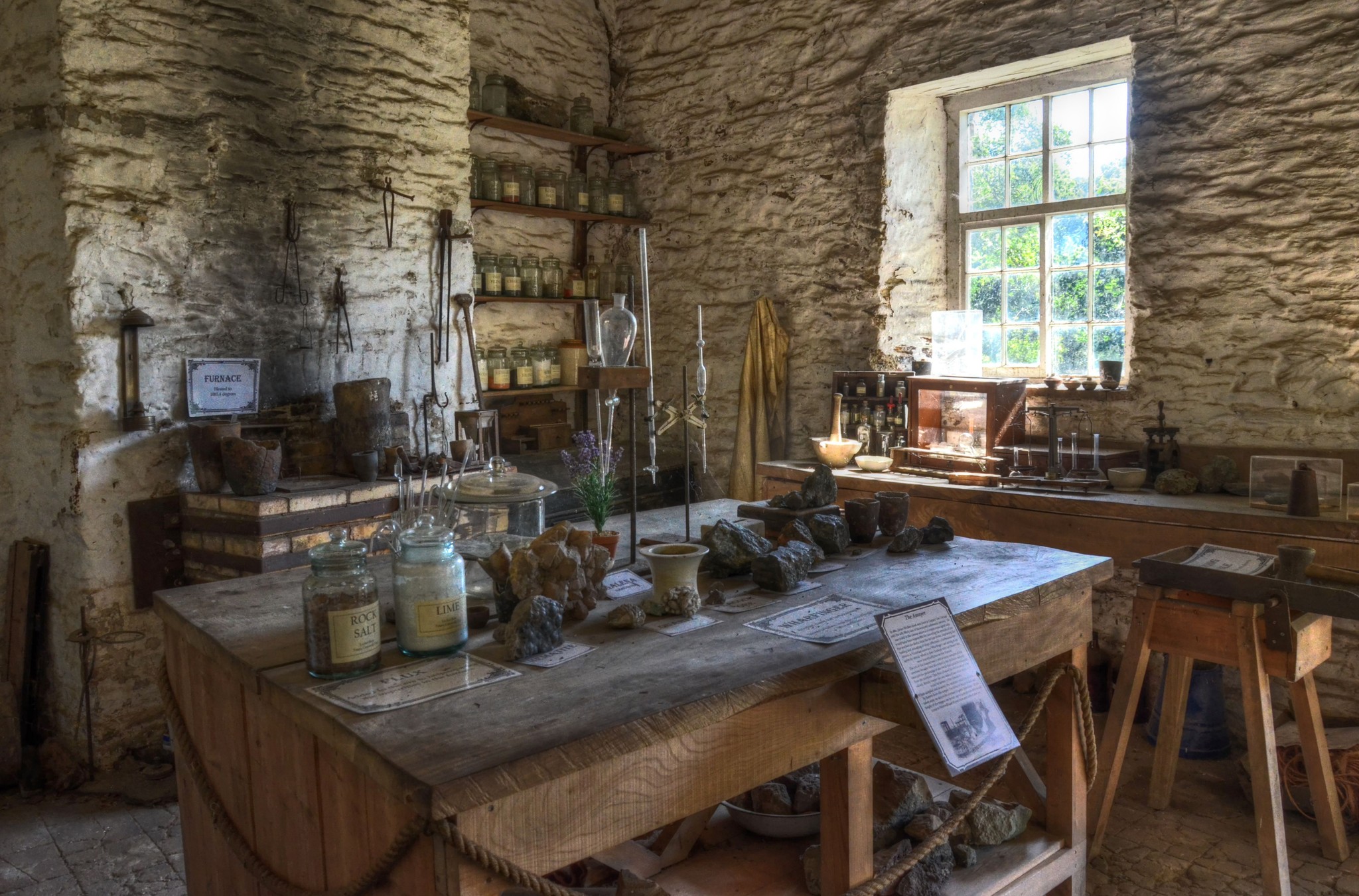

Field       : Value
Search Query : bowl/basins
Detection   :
[1082,377,1097,390]
[720,800,821,839]
[1063,376,1082,389]
[855,456,894,472]
[1107,467,1147,491]
[1101,376,1119,389]
[1044,373,1062,388]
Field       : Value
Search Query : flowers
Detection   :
[558,428,624,531]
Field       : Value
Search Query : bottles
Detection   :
[1008,446,1024,486]
[475,338,588,391]
[468,67,593,135]
[959,432,986,472]
[1086,434,1106,491]
[469,153,638,217]
[840,374,906,458]
[301,526,382,678]
[1057,438,1068,479]
[472,249,633,300]
[599,293,637,368]
[392,514,468,658]
[433,456,558,602]
[1064,432,1085,489]
[1088,631,1150,724]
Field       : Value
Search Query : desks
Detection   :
[152,497,1114,896]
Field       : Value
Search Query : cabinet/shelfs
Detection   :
[837,369,915,465]
[470,107,660,455]
[757,461,1358,620]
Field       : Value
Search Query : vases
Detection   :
[579,531,620,558]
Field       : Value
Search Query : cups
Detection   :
[467,606,490,629]
[1100,360,1123,387]
